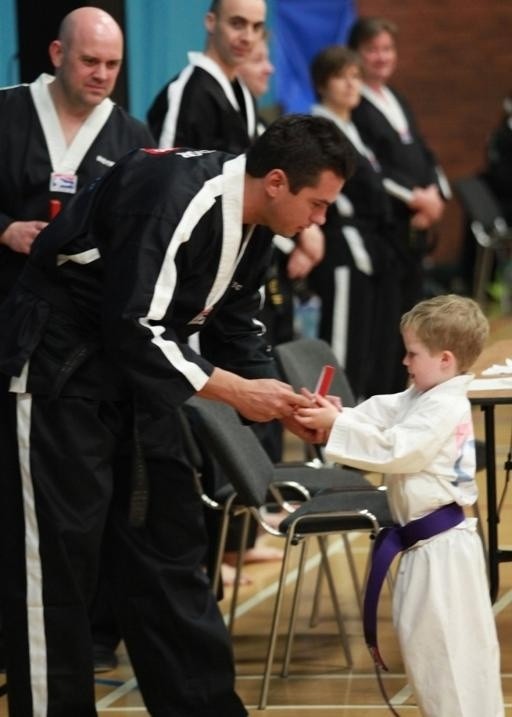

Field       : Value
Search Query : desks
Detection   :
[465,339,512,608]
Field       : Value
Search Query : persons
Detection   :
[294,293,505,717]
[347,15,454,390]
[0,113,359,716]
[232,31,326,347]
[145,0,284,585]
[454,95,512,306]
[0,6,156,669]
[308,44,446,473]
